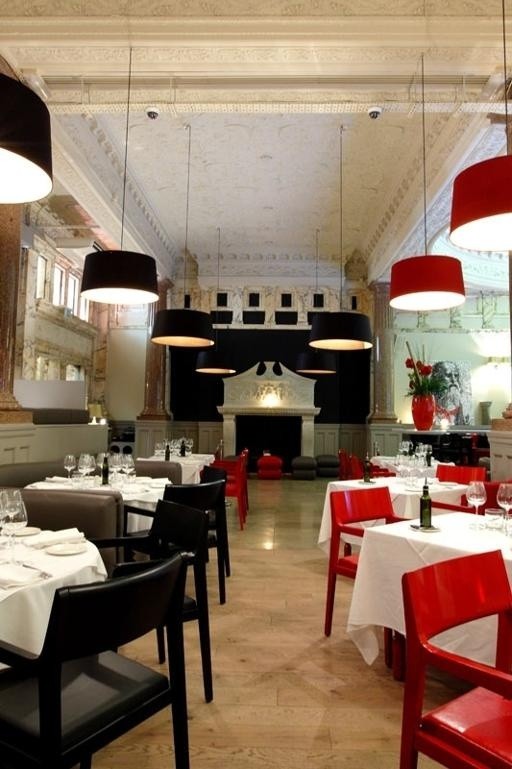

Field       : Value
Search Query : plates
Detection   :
[48,543,87,555]
[17,526,40,535]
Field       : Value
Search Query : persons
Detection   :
[431,361,470,425]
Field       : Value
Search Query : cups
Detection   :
[483,509,512,543]
[480,401,493,425]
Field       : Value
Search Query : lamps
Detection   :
[256,360,283,376]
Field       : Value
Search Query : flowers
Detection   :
[402,340,450,398]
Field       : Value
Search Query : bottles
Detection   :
[164,445,171,462]
[419,477,434,527]
[362,452,372,483]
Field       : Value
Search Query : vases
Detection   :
[411,395,436,431]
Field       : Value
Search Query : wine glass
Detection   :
[0,487,28,576]
[64,453,137,491]
[155,438,194,458]
[497,483,512,519]
[393,441,434,484]
[465,480,487,530]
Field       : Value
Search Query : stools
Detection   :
[257,454,338,480]
